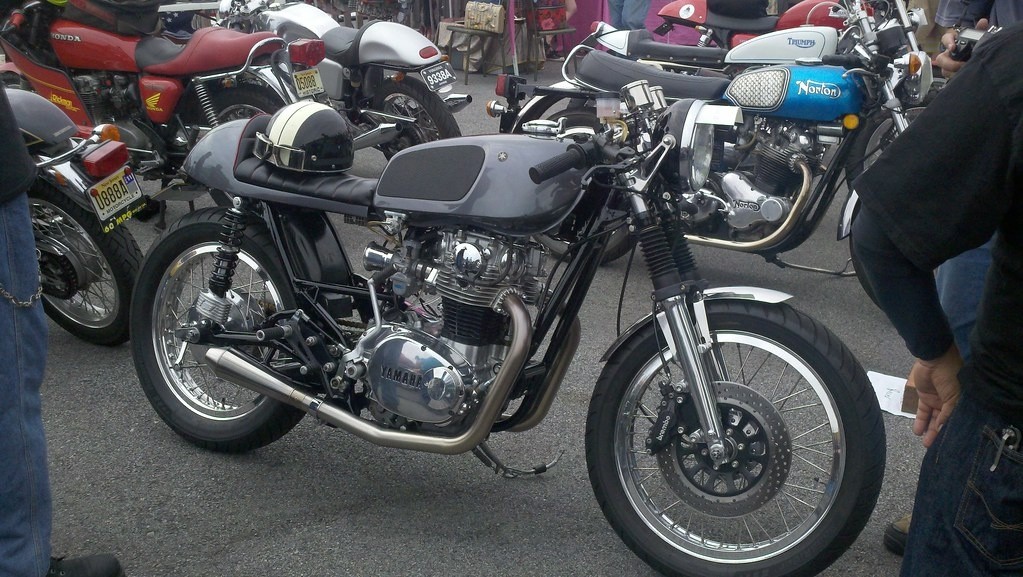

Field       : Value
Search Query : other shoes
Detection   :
[45,554,123,577]
[884,512,915,556]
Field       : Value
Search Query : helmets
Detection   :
[261,101,354,173]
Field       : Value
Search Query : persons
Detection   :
[544,0,577,61]
[608,0,650,61]
[850,0,1023,577]
[0,79,117,577]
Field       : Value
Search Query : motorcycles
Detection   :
[0,0,947,347]
[129,76,886,577]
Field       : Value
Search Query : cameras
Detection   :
[948,24,988,62]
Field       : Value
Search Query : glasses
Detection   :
[253,132,306,172]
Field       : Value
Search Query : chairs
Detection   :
[523,0,578,81]
[446,0,510,85]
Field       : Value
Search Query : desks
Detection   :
[434,17,547,75]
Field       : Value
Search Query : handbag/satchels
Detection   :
[466,2,506,34]
